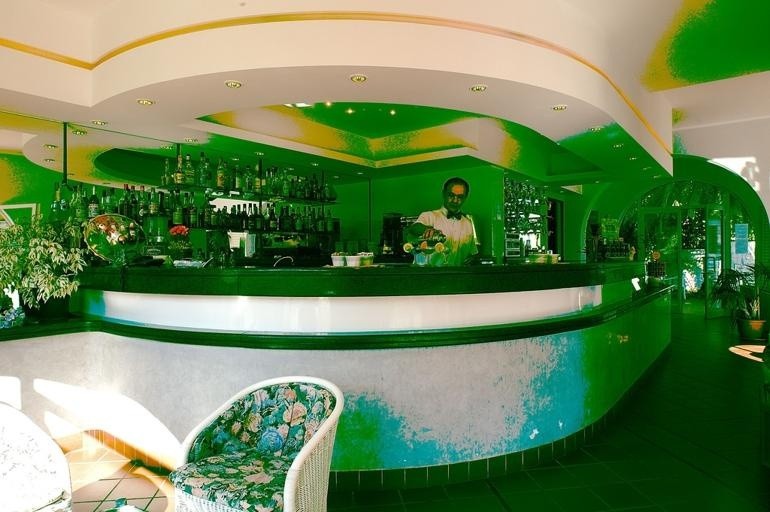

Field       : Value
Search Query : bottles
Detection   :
[189,192,197,227]
[148,187,159,216]
[137,185,149,225]
[162,158,173,183]
[158,191,165,216]
[603,237,628,257]
[242,204,248,230]
[101,187,118,214]
[216,159,330,201]
[119,184,132,216]
[196,152,208,186]
[71,182,88,218]
[89,186,100,219]
[201,205,237,231]
[165,197,172,226]
[206,158,213,186]
[237,205,243,231]
[520,238,545,256]
[184,193,191,228]
[129,186,139,221]
[254,202,333,234]
[418,230,446,246]
[172,189,183,226]
[173,154,185,184]
[248,203,254,230]
[184,155,195,185]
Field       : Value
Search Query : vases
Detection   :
[168,239,193,264]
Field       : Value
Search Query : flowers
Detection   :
[169,226,189,240]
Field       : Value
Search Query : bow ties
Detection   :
[446,210,462,220]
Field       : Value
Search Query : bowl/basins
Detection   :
[332,256,347,267]
[345,255,375,267]
[529,253,549,265]
[551,253,560,265]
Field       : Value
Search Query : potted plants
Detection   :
[708,263,767,343]
[262,232,300,268]
[331,242,345,266]
[357,238,374,266]
[345,239,360,267]
[231,247,247,268]
[15,216,91,324]
[208,229,230,266]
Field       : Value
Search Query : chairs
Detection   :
[168,376,345,512]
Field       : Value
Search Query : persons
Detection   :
[407,178,479,266]
